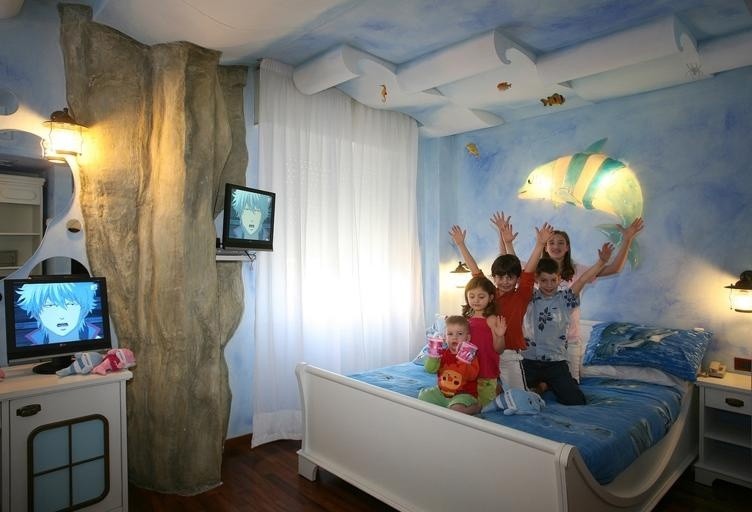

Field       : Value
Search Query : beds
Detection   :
[294,356,702,512]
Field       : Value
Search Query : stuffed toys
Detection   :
[481,389,545,417]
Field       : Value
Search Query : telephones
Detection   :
[701,361,726,378]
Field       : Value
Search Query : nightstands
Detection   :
[693,371,752,486]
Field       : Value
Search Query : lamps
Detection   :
[41,107,83,165]
[724,269,752,313]
[451,262,473,289]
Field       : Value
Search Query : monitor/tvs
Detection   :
[221,183,276,252]
[4,277,112,375]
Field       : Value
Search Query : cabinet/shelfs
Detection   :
[0,172,46,279]
[0,364,133,512]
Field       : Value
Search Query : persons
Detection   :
[490,211,645,386]
[417,316,481,415]
[442,276,507,409]
[11,281,103,346]
[499,224,615,405]
[230,187,273,242]
[448,222,554,392]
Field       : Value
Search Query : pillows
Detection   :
[579,320,690,394]
[583,322,713,382]
[414,313,447,366]
